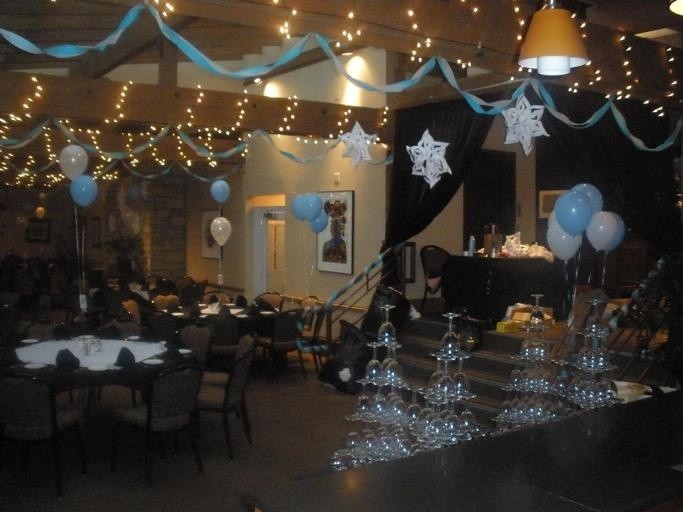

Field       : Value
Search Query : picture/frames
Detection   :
[199,209,223,260]
[313,189,354,275]
[25,218,50,243]
[539,190,571,219]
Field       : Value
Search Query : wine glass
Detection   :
[327,290,616,470]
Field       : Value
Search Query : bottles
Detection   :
[486,225,502,260]
[467,235,476,258]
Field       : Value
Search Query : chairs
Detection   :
[0,252,342,499]
[420,245,450,313]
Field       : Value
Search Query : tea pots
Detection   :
[77,334,101,356]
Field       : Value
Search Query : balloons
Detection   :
[545,183,625,265]
[59,144,88,181]
[210,216,232,246]
[35,207,46,219]
[210,180,231,204]
[291,192,329,233]
[70,174,98,208]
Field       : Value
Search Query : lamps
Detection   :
[517,0,589,77]
